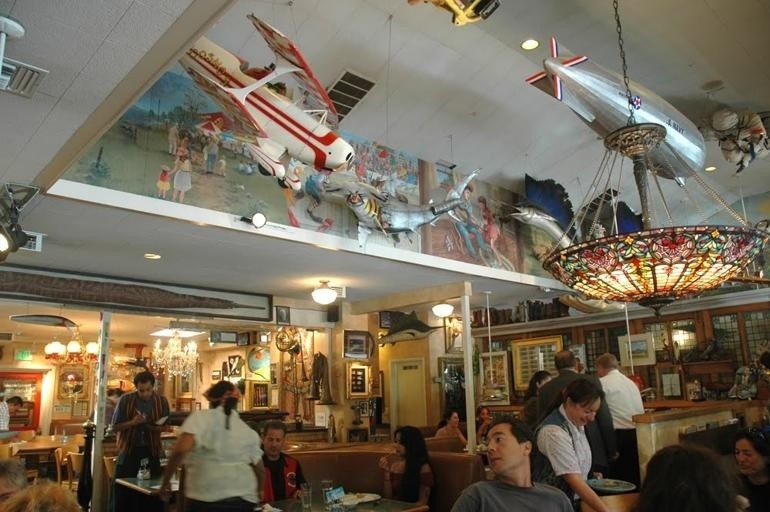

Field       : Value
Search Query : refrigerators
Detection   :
[0,372,42,434]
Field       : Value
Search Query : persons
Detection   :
[261,421,308,504]
[89,389,125,428]
[158,381,265,512]
[0,482,82,512]
[435,349,645,512]
[636,419,770,512]
[0,457,27,504]
[449,185,516,272]
[758,350,770,413]
[245,420,261,436]
[154,120,258,202]
[378,426,434,507]
[109,371,170,512]
[0,396,23,440]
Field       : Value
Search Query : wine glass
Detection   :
[587,478,636,493]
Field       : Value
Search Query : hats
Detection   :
[176,147,188,157]
[161,164,170,171]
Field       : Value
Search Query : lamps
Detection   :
[148,330,201,381]
[431,298,454,318]
[541,1,769,320]
[44,326,99,365]
[233,210,267,229]
[0,182,40,263]
[311,279,338,305]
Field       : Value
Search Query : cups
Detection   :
[319,481,334,510]
[300,482,312,509]
[50,425,68,443]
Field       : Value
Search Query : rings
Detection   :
[383,461,385,464]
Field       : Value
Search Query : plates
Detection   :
[345,493,382,503]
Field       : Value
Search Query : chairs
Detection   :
[51,416,88,435]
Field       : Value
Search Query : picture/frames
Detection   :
[617,331,686,400]
[341,329,372,401]
[227,342,280,411]
[1,263,273,321]
[276,305,291,326]
[173,358,201,412]
[438,314,565,425]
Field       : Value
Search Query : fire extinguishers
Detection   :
[369,397,376,425]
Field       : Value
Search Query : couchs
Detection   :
[286,423,487,511]
